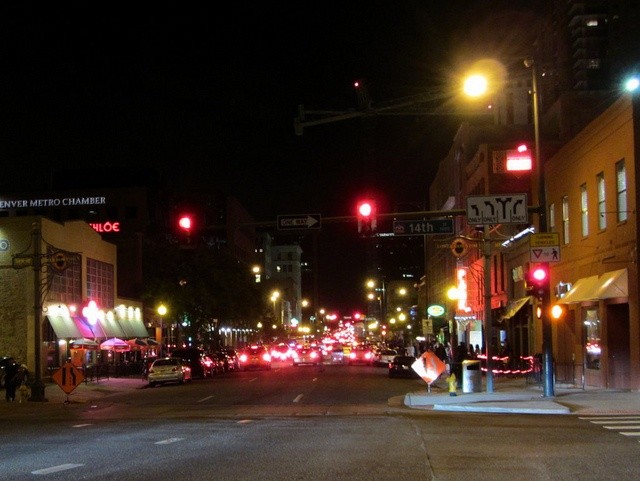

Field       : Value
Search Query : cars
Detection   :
[239,344,271,371]
[310,325,381,364]
[387,355,420,378]
[171,347,215,378]
[273,343,292,361]
[225,350,239,369]
[292,348,318,366]
[211,352,235,374]
[349,344,381,366]
[148,357,191,387]
[378,349,399,366]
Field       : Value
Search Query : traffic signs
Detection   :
[391,217,455,236]
[276,214,322,232]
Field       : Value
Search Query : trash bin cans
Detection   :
[461,360,481,393]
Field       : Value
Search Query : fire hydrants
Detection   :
[445,373,457,395]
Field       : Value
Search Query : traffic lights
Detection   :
[360,200,372,235]
[354,311,360,322]
[179,214,192,245]
[532,266,548,301]
[331,312,337,320]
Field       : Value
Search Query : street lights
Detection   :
[158,300,167,357]
[369,294,382,335]
[270,291,278,342]
[388,317,395,324]
[294,298,308,318]
[399,287,411,346]
[367,280,386,342]
[257,322,262,344]
[398,312,405,321]
[316,308,324,322]
[463,58,553,397]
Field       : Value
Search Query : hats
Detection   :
[19,364,28,370]
